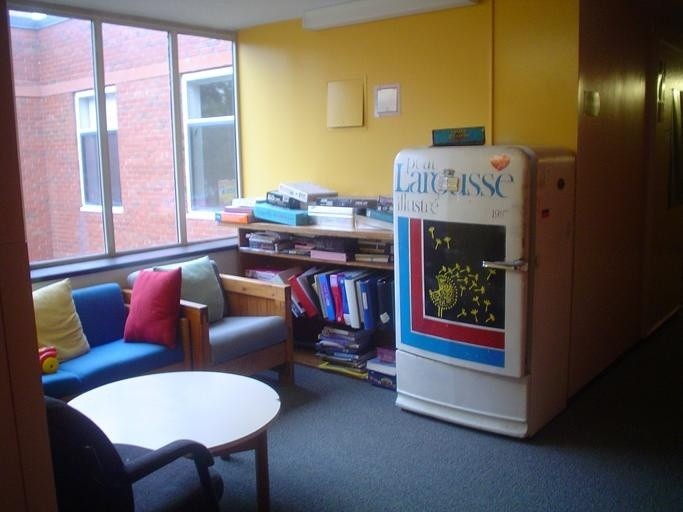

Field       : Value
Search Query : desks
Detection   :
[67,371,281,511]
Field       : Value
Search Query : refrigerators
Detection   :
[393,143,576,440]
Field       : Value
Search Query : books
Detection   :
[245,232,394,263]
[221,183,393,230]
[245,268,396,391]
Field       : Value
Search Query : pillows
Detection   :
[123,267,182,349]
[32,277,91,363]
[153,255,225,323]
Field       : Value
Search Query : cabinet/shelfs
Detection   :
[237,223,394,380]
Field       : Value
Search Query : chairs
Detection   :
[122,259,295,385]
[44,395,224,512]
[32,283,193,402]
